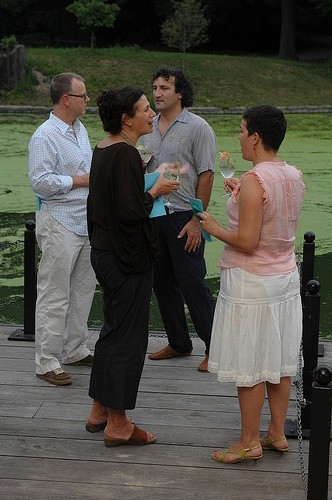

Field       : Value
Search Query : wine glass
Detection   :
[161,169,181,206]
[219,158,237,197]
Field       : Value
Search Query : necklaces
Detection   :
[119,131,135,146]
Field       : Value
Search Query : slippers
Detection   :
[104,425,157,448]
[86,416,135,433]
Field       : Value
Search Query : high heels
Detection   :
[260,434,288,455]
[211,442,263,464]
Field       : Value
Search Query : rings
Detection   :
[200,220,202,222]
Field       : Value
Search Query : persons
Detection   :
[139,67,216,373]
[28,72,95,385]
[197,105,303,465]
[87,86,181,447]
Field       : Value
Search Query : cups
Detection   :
[139,152,152,168]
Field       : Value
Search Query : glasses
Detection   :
[67,93,88,100]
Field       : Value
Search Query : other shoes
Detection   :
[148,345,193,360]
[199,355,210,373]
[36,368,72,386]
[63,354,94,366]
